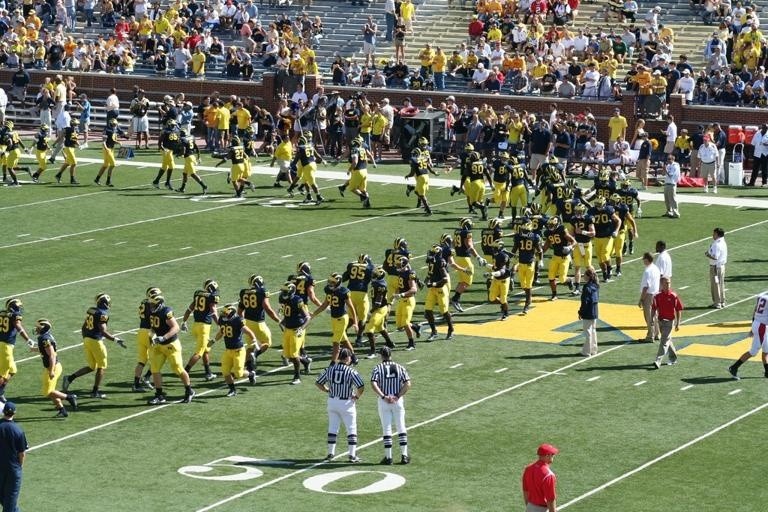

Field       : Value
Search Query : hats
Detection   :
[537,444,558,455]
[78,94,87,99]
[381,96,456,104]
[2,402,16,412]
[380,347,391,357]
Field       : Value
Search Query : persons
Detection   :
[650,278,683,369]
[662,154,681,219]
[726,290,767,379]
[578,270,601,357]
[0,0,767,214]
[313,347,366,464]
[476,179,641,318]
[338,217,486,356]
[639,252,661,342]
[130,261,359,404]
[705,228,727,309]
[370,345,411,466]
[655,241,672,291]
[0,292,128,418]
[0,402,29,512]
[521,443,561,512]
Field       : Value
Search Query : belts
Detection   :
[330,395,348,400]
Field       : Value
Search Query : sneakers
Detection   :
[325,454,336,462]
[55,174,61,183]
[281,350,313,385]
[402,455,411,463]
[227,173,255,198]
[523,306,528,314]
[53,376,79,418]
[485,197,489,205]
[460,203,560,255]
[274,182,324,205]
[380,457,393,465]
[502,312,509,319]
[450,297,464,312]
[451,186,456,195]
[623,247,633,255]
[92,392,106,398]
[338,186,346,197]
[106,181,114,186]
[207,352,257,396]
[71,178,80,183]
[550,295,557,300]
[3,167,38,186]
[133,377,166,404]
[407,185,433,216]
[568,279,582,295]
[153,181,184,193]
[349,455,362,462]
[405,324,454,351]
[360,191,370,206]
[183,389,195,403]
[729,366,740,379]
[94,179,101,185]
[604,268,621,282]
[352,335,377,364]
[203,185,208,196]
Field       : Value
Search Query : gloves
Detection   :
[26,339,38,349]
[154,336,164,343]
[114,337,127,349]
[181,320,188,332]
[207,339,214,347]
[251,339,260,351]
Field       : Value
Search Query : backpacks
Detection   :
[136,101,147,116]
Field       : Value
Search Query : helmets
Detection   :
[165,118,188,136]
[3,121,13,130]
[297,130,312,144]
[352,137,362,147]
[70,117,79,127]
[204,279,219,293]
[248,274,264,289]
[41,124,51,136]
[280,254,386,301]
[108,118,119,128]
[465,143,480,161]
[418,137,430,145]
[146,287,165,313]
[499,151,518,165]
[568,169,632,219]
[96,294,111,309]
[338,348,352,359]
[6,299,24,316]
[32,318,52,337]
[223,305,237,319]
[393,233,454,272]
[549,155,558,163]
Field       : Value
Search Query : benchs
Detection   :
[0,0,768,179]
